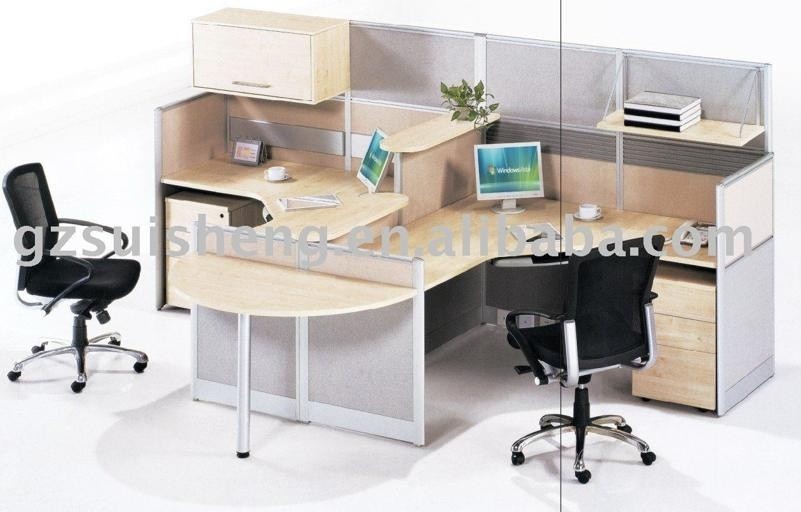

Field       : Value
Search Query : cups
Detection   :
[264,166,286,179]
[579,204,601,218]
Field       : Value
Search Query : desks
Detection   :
[151,151,717,466]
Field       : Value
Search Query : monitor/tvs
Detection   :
[472,142,546,216]
[356,126,393,199]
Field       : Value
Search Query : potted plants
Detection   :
[440,79,501,127]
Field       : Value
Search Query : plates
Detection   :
[574,212,601,222]
[264,175,288,182]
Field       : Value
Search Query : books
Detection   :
[623,91,702,132]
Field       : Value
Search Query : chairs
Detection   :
[482,234,672,486]
[0,158,147,394]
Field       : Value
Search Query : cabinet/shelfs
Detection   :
[188,5,349,107]
